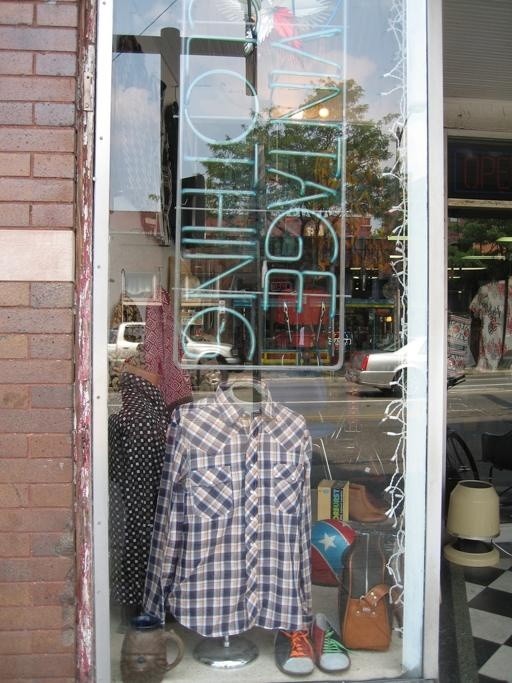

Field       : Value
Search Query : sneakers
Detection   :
[272,628,315,676]
[312,611,353,673]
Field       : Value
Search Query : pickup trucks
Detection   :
[108,320,245,391]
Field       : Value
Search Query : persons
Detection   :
[141,379,314,636]
[466,274,512,369]
[110,366,169,606]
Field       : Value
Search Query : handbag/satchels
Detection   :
[339,534,396,652]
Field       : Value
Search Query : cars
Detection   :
[345,338,406,395]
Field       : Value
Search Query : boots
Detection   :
[349,487,385,523]
[353,481,386,515]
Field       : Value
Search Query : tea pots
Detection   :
[119,615,185,683]
